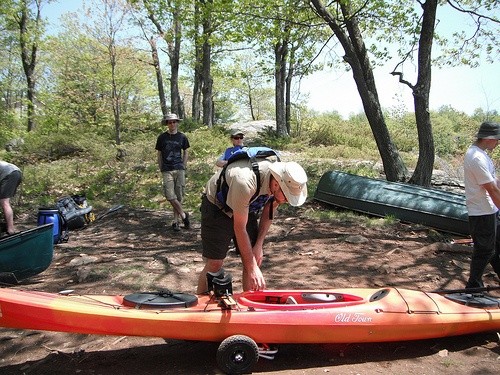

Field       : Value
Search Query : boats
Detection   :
[312,170,472,238]
[0,221,55,287]
[0,283,500,344]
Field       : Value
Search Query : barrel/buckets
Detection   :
[38,208,61,243]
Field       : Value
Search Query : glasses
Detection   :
[169,121,176,124]
[232,136,243,140]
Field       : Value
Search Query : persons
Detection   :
[156,114,191,229]
[197,158,308,294]
[464,122,500,287]
[216,133,259,256]
[0,161,22,235]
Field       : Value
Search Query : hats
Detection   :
[268,161,308,206]
[161,114,183,125]
[473,122,500,139]
[231,129,244,135]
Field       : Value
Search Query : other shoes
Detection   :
[182,212,189,229]
[172,223,181,230]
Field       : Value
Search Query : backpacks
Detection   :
[55,195,93,230]
